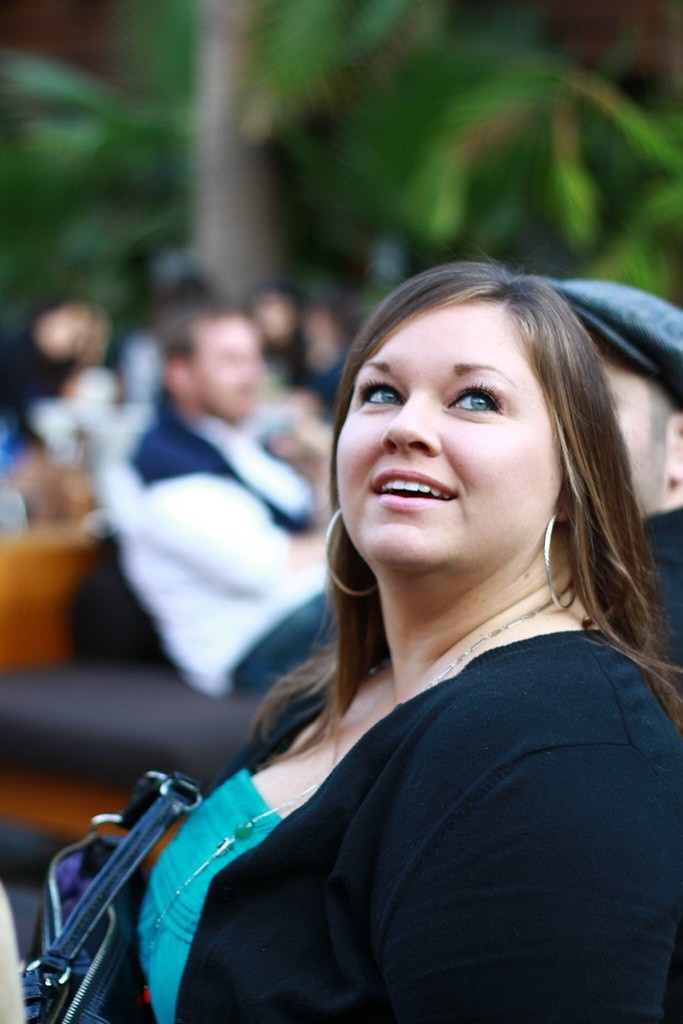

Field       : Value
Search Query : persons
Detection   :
[0,261,683,1024]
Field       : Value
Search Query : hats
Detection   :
[545,279,683,410]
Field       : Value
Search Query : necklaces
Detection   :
[154,574,572,932]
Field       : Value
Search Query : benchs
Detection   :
[0,521,321,887]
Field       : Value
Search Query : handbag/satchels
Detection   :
[21,769,201,1024]
